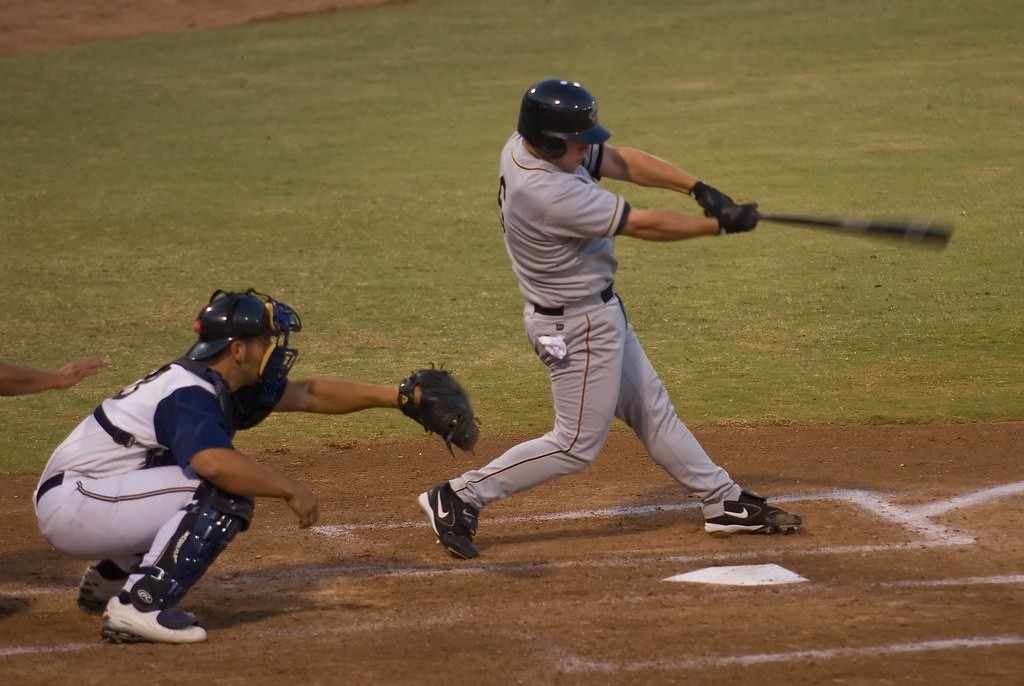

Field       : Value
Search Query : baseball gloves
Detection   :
[398,360,480,456]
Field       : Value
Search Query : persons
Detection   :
[34,287,478,643]
[0,357,113,397]
[417,80,802,561]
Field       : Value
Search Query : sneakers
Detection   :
[76,565,131,608]
[417,482,480,559]
[100,596,207,644]
[705,490,803,535]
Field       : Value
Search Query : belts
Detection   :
[532,283,614,316]
[36,473,64,506]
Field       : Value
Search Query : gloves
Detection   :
[689,180,735,217]
[716,202,758,235]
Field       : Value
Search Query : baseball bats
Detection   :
[756,212,956,251]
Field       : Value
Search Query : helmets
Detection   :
[185,293,270,360]
[517,79,612,147]
